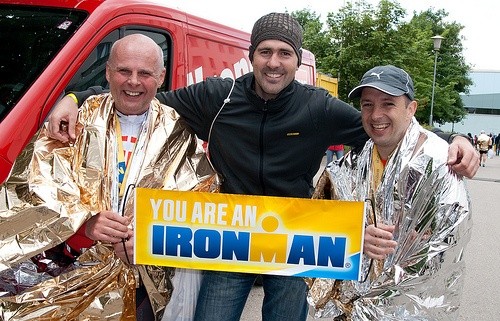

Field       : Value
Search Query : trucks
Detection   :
[317,71,338,101]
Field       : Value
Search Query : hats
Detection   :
[348,65,414,101]
[249,12,303,68]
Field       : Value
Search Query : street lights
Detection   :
[428,35,444,129]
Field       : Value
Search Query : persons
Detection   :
[0,32,219,321]
[45,13,482,321]
[301,64,472,321]
[327,145,343,163]
[465,130,500,167]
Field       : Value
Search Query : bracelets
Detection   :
[62,94,77,104]
[449,132,458,143]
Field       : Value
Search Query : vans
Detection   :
[0,0,316,254]
